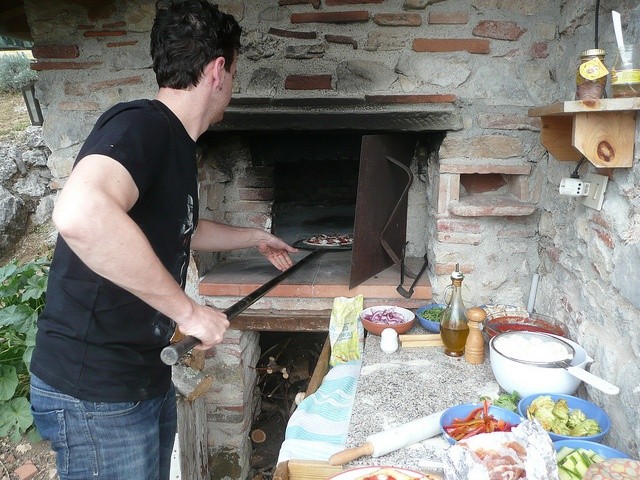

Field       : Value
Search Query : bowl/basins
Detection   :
[415,304,446,333]
[440,263,469,356]
[360,306,415,336]
[517,393,610,442]
[554,440,632,480]
[483,311,568,339]
[439,403,525,446]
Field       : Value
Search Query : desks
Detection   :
[272,317,504,480]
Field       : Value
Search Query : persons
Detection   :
[27,1,300,480]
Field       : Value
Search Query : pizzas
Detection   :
[302,234,353,247]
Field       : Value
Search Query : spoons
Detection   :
[493,333,621,397]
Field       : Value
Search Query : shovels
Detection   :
[160,234,352,365]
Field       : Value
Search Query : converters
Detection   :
[559,177,590,198]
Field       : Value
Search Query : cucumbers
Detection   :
[556,447,604,480]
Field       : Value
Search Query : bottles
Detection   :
[465,307,486,364]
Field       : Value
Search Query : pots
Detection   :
[489,332,594,399]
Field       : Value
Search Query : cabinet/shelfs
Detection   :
[527,97,640,170]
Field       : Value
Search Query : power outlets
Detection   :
[577,173,609,211]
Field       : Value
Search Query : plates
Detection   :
[328,466,440,480]
